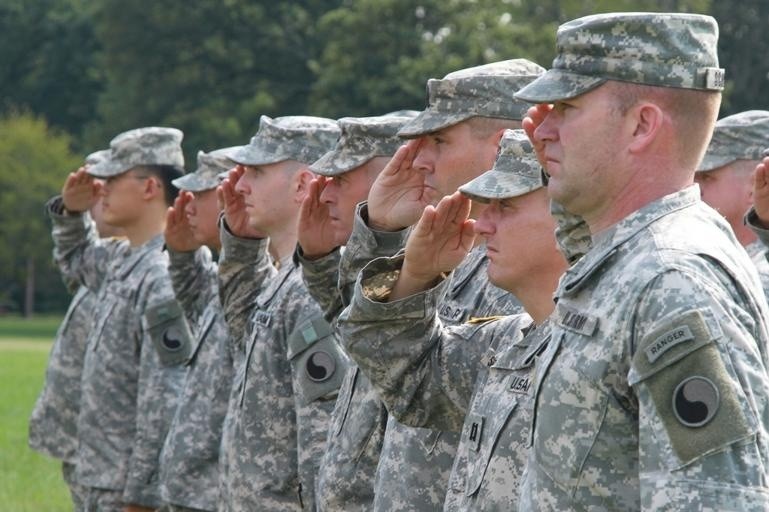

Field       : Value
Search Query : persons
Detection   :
[694,110,769,306]
[292,107,423,510]
[512,11,769,512]
[336,58,545,510]
[29,148,124,510]
[217,113,344,511]
[45,126,210,512]
[163,143,279,510]
[337,124,570,510]
[744,151,769,303]
